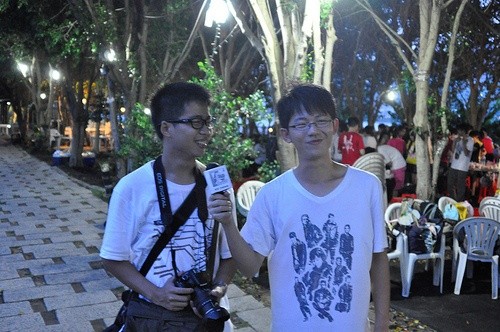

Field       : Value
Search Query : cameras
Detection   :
[174,267,230,323]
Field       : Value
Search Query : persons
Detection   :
[207,84,391,332]
[99,82,239,332]
[331,117,417,199]
[437,123,500,202]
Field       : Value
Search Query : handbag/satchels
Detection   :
[124,296,207,332]
[102,303,129,332]
[408,217,441,254]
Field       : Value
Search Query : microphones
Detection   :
[204,162,232,212]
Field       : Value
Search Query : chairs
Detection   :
[236,180,266,278]
[0,124,111,153]
[352,152,500,300]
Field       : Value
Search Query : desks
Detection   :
[467,169,500,206]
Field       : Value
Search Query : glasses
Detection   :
[288,116,332,131]
[166,116,216,129]
